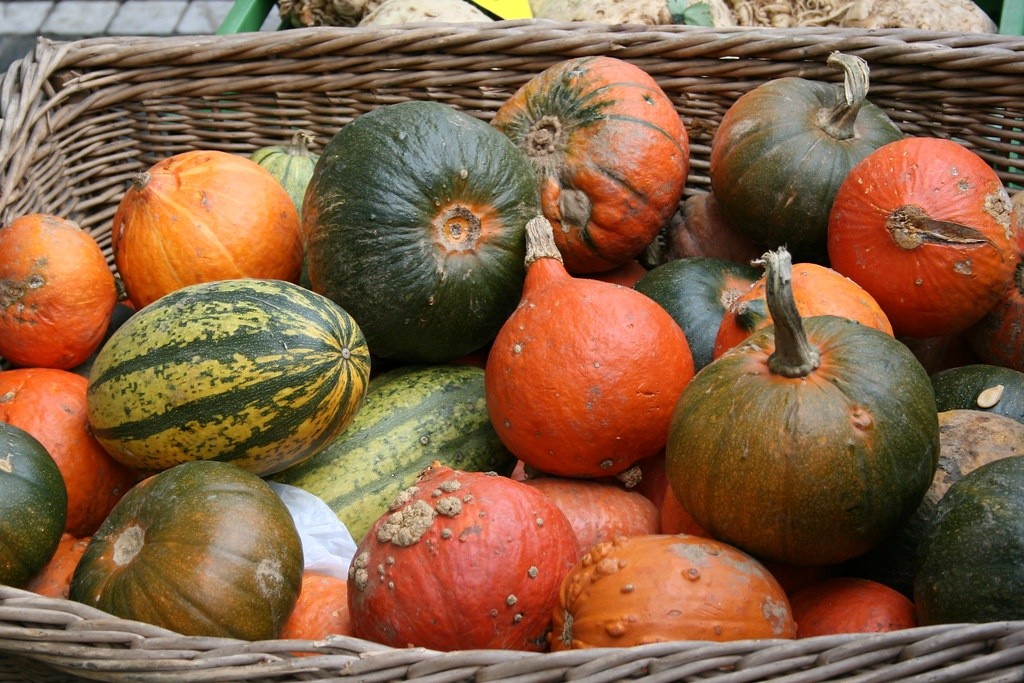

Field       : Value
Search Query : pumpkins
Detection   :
[0,53,1024,647]
